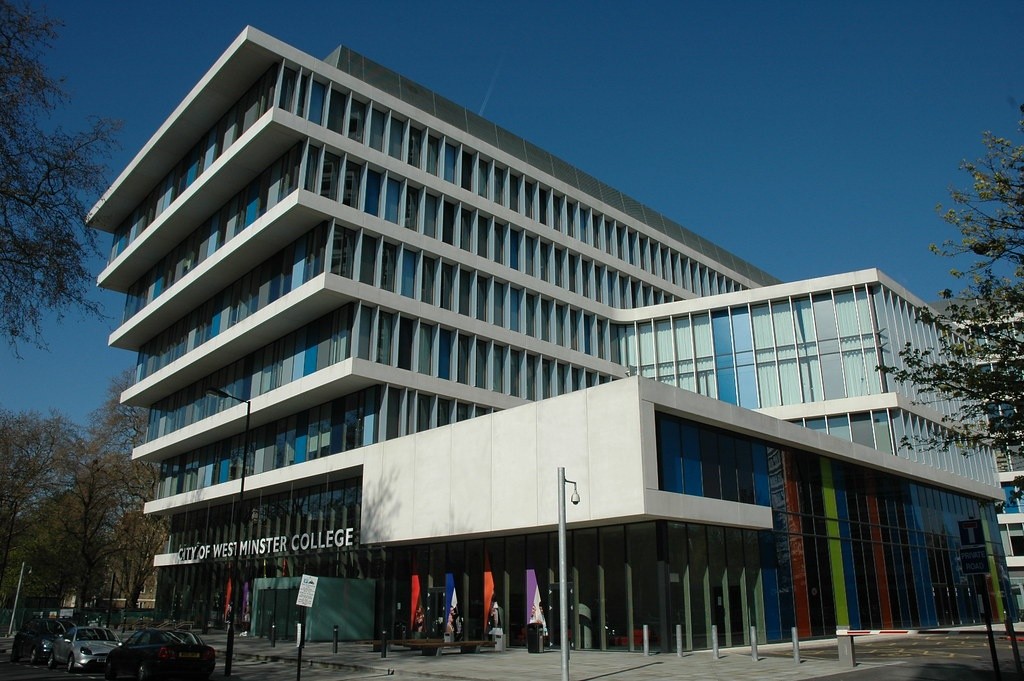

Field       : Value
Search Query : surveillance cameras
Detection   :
[571,493,581,505]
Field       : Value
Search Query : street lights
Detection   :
[7,562,33,635]
[556,466,580,681]
[105,564,117,629]
[202,389,251,677]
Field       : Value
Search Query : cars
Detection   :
[9,619,78,666]
[105,629,217,681]
[47,626,122,675]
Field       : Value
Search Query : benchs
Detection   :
[370,638,497,657]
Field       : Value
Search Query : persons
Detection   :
[447,606,463,635]
[489,602,502,629]
[415,607,425,632]
[530,602,548,635]
[226,610,230,621]
